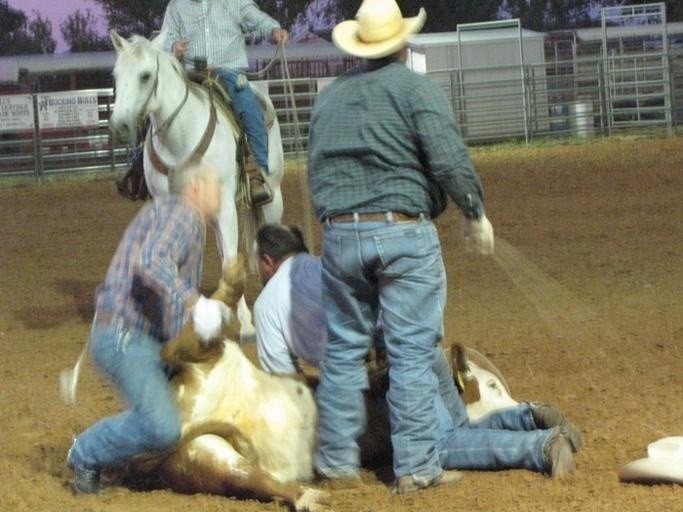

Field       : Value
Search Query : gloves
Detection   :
[461,214,494,255]
[192,294,231,342]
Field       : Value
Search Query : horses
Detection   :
[102,25,287,347]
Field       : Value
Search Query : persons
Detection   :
[150,1,289,204]
[304,0,495,496]
[250,222,583,481]
[60,154,235,498]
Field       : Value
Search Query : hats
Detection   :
[330,0,427,59]
[618,436,682,485]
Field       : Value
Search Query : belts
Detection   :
[333,212,416,223]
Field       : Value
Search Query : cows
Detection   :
[95,253,522,512]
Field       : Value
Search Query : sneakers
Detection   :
[74,464,100,497]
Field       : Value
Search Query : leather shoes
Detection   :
[396,471,463,495]
[250,178,269,203]
[543,425,582,482]
[529,401,582,453]
